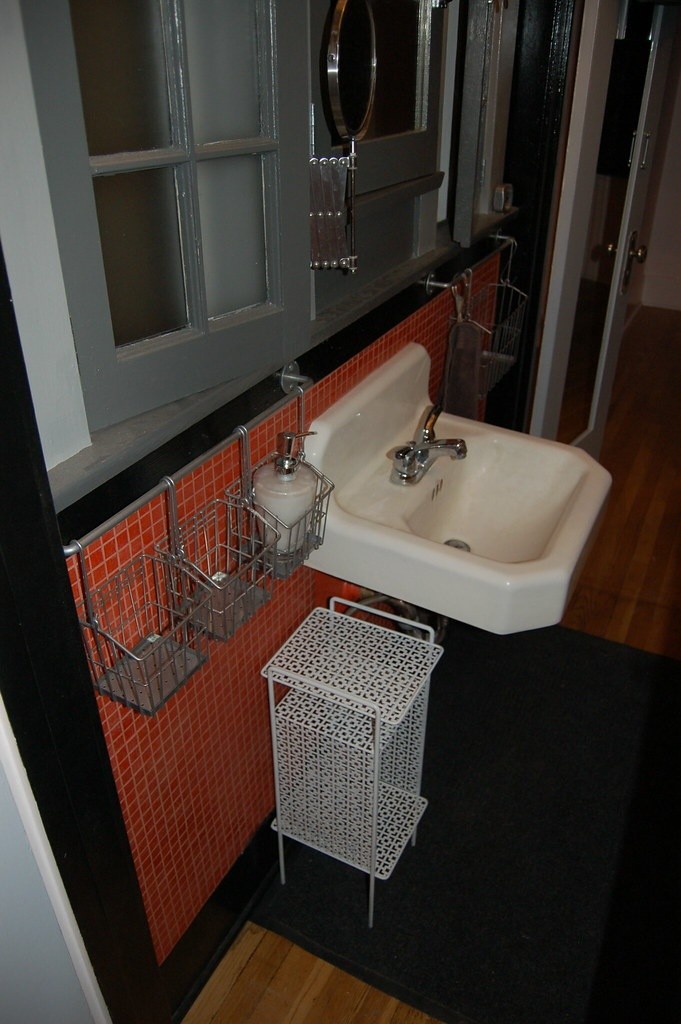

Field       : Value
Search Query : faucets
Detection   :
[389,405,469,486]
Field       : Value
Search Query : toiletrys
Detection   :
[254,429,320,556]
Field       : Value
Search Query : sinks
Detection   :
[334,410,612,636]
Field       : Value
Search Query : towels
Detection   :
[438,321,484,422]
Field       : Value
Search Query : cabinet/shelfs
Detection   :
[442,282,530,426]
[70,385,335,714]
[262,598,444,928]
